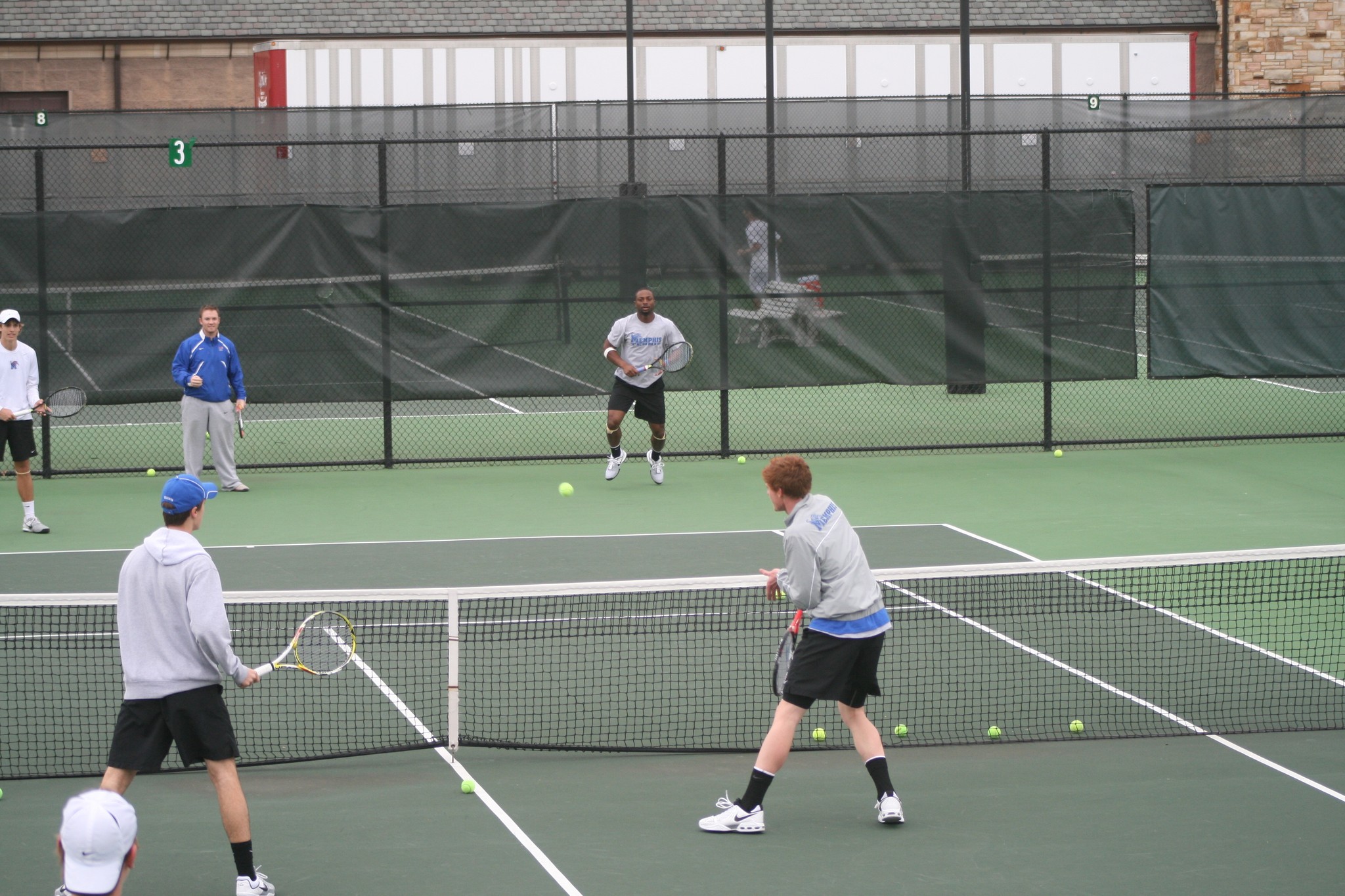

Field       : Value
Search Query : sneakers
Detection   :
[605,448,626,481]
[646,449,665,484]
[23,515,50,533]
[232,484,249,492]
[874,790,905,823]
[236,866,275,896]
[699,790,765,832]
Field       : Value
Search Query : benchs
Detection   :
[727,281,848,348]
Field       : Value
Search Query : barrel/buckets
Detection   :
[798,274,824,311]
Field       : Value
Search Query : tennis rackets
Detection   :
[772,610,803,696]
[251,610,356,677]
[635,341,694,373]
[236,404,244,439]
[11,386,87,418]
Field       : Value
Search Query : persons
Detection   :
[54,790,138,896]
[169,302,250,492]
[737,203,781,300]
[697,456,905,834]
[97,474,275,896]
[601,289,687,484]
[0,310,53,533]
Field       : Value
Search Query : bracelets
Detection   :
[665,360,670,366]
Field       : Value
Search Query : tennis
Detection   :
[147,469,155,477]
[559,482,574,497]
[812,728,826,741]
[206,432,210,439]
[1070,720,1084,733]
[988,726,1001,739]
[894,724,908,737]
[1054,449,1062,457]
[775,590,786,600]
[737,456,746,464]
[461,780,475,793]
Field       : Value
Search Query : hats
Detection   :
[161,474,218,515]
[0,309,21,324]
[60,790,137,895]
